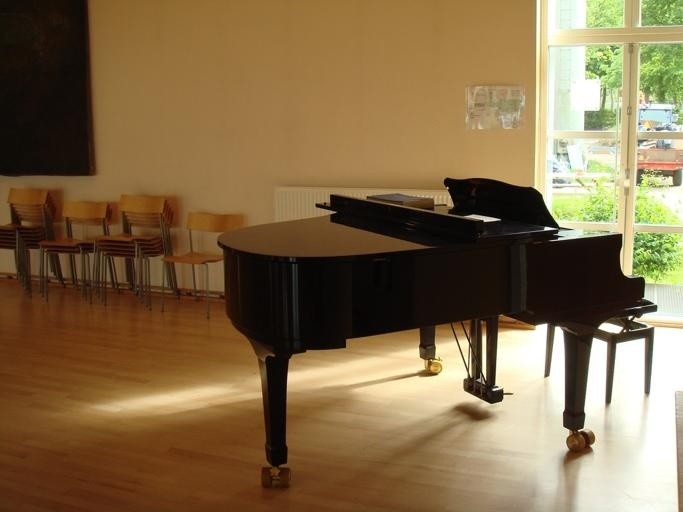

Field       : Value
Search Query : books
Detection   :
[367,193,434,209]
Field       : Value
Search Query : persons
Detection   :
[639,92,647,104]
[648,95,655,106]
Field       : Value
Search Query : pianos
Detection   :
[216,178,645,490]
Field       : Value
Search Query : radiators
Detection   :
[273,186,456,223]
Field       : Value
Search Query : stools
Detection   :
[545,318,657,405]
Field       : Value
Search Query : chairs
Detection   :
[0,186,244,321]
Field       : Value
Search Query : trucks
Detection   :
[620,102,683,188]
